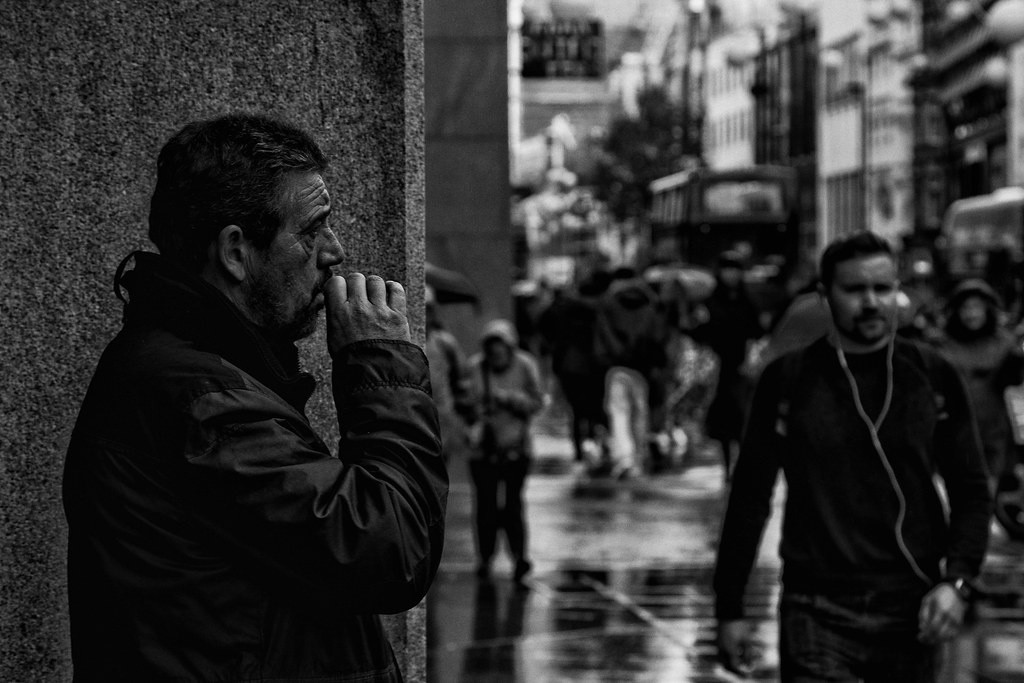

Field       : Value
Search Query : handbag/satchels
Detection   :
[482,423,530,483]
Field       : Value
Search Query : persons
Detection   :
[61,114,449,683]
[703,230,1023,683]
[432,318,545,610]
[512,261,717,485]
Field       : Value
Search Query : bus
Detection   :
[648,165,799,341]
[937,186,1023,336]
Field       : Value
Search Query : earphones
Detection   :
[822,296,830,312]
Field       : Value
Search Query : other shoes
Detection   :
[513,560,530,580]
[476,564,491,578]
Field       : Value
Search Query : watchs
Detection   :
[943,575,979,605]
